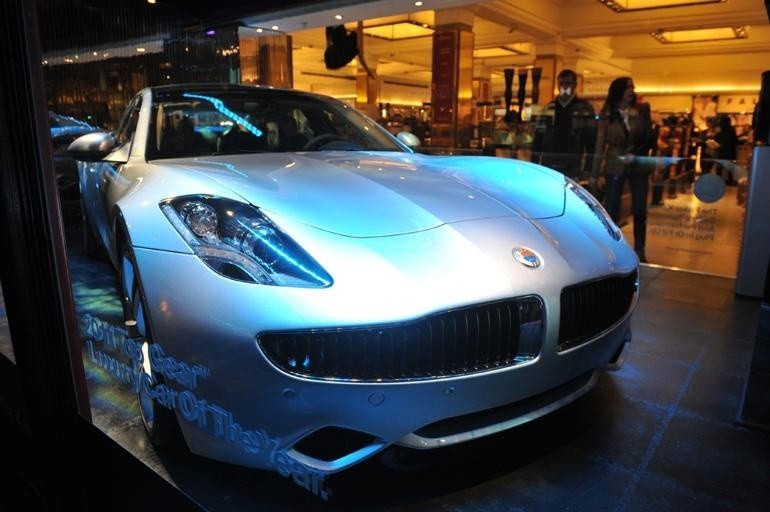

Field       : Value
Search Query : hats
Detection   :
[48,110,110,213]
[65,81,642,480]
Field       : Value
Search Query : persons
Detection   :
[531,67,594,183]
[652,114,737,187]
[497,109,535,144]
[592,76,652,266]
